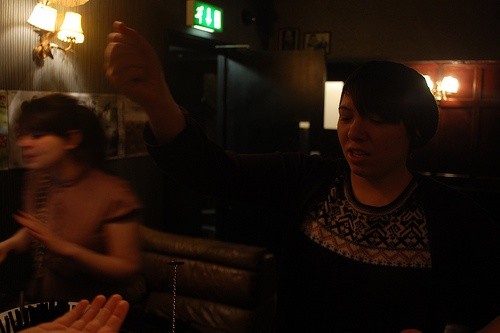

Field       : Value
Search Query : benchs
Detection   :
[122,227,260,333]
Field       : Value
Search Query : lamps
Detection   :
[26,3,84,68]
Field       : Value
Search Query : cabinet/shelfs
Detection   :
[401,61,500,192]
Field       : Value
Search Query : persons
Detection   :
[0,93,147,308]
[103,21,500,333]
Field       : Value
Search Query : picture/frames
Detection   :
[277,27,301,51]
[302,31,332,50]
